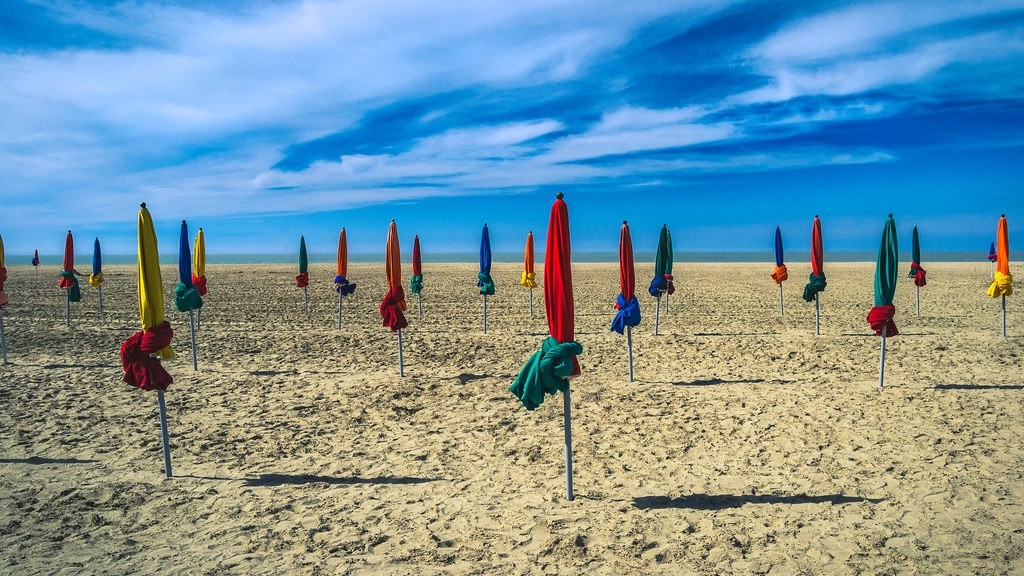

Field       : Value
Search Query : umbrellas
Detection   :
[610,220,640,382]
[0,234,9,370]
[296,236,310,322]
[770,225,788,315]
[173,220,208,371]
[477,222,495,335]
[867,214,901,387]
[409,235,426,315]
[908,223,926,317]
[89,238,105,311]
[985,214,1015,337]
[58,229,81,326]
[508,192,583,502]
[334,226,357,330]
[380,218,407,377]
[120,202,176,478]
[32,249,40,278]
[802,214,827,336]
[648,224,675,335]
